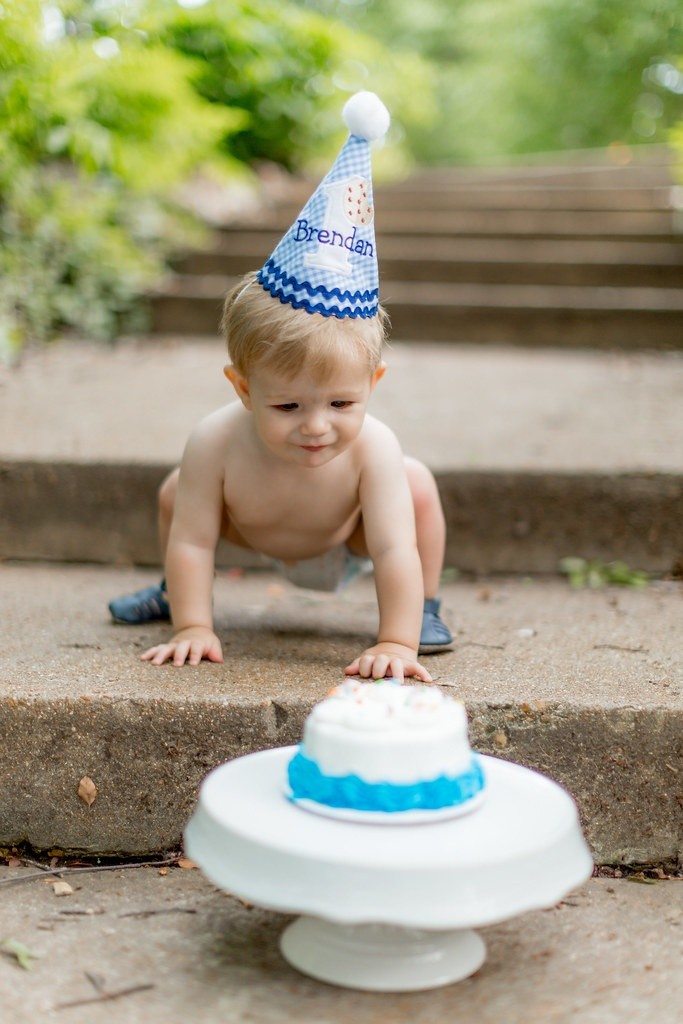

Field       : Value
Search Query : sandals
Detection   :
[418,600,454,655]
[108,579,170,624]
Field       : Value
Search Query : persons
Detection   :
[109,272,453,682]
